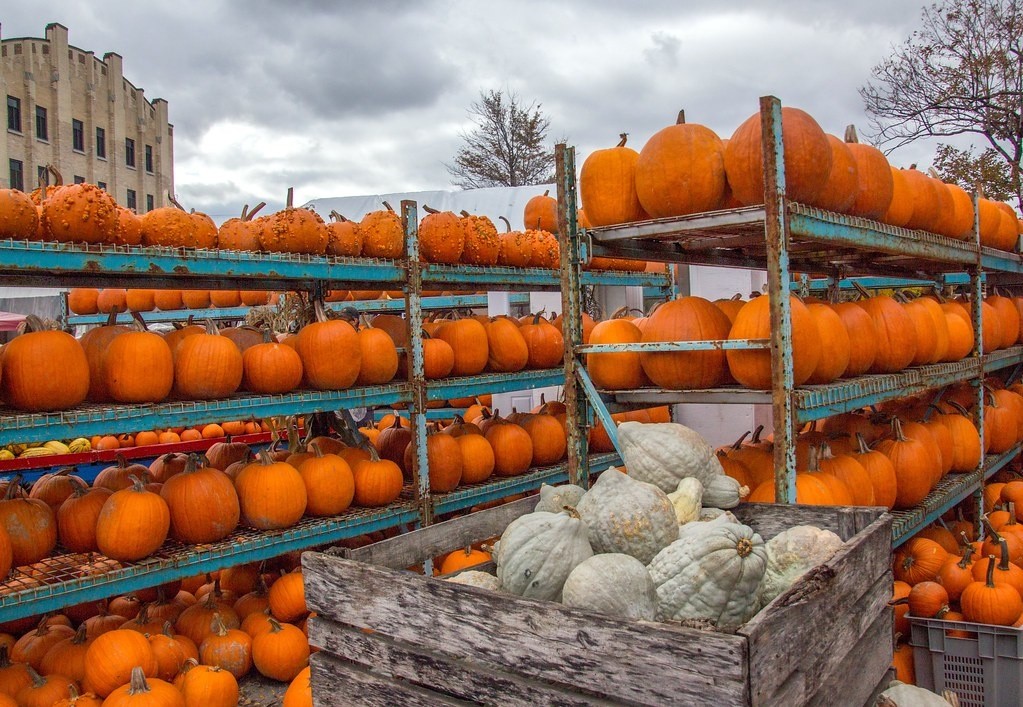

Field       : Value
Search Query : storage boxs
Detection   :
[300,492,897,707]
[903,609,1022,707]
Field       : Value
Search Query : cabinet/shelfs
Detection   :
[0,200,677,707]
[554,97,1023,548]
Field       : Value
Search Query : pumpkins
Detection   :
[0,104,1023,705]
